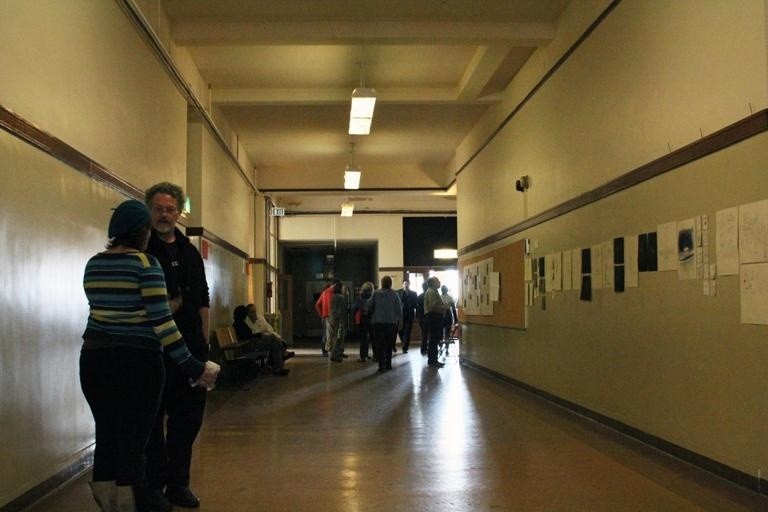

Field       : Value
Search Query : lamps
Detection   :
[348,65,377,136]
[341,192,354,218]
[343,142,361,189]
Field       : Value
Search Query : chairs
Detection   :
[210,327,267,388]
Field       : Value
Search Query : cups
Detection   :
[200,360,219,389]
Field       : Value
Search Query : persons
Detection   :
[79,198,219,512]
[245,304,294,360]
[314,276,458,371]
[232,305,290,376]
[142,181,210,512]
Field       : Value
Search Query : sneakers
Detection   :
[167,491,200,508]
